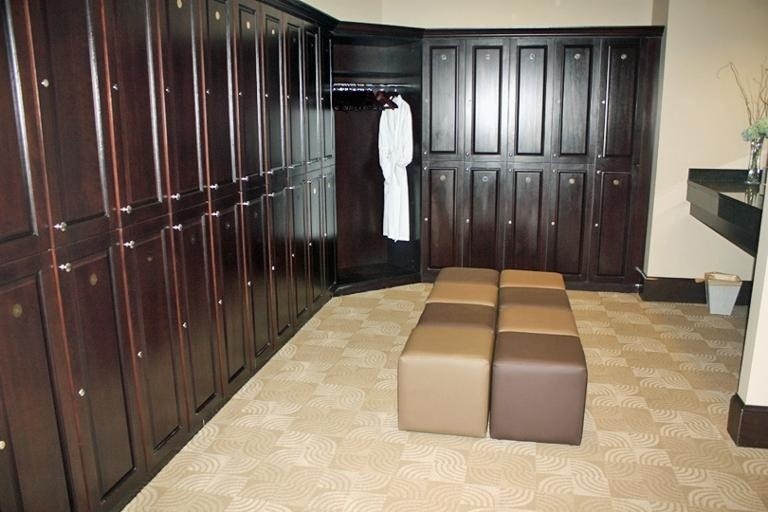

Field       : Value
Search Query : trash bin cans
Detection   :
[705,273,743,315]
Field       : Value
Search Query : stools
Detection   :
[396,265,589,445]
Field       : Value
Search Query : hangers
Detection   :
[334,80,412,113]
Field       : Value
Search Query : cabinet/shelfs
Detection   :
[417,21,667,295]
[1,0,336,512]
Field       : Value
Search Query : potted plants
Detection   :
[724,59,765,184]
